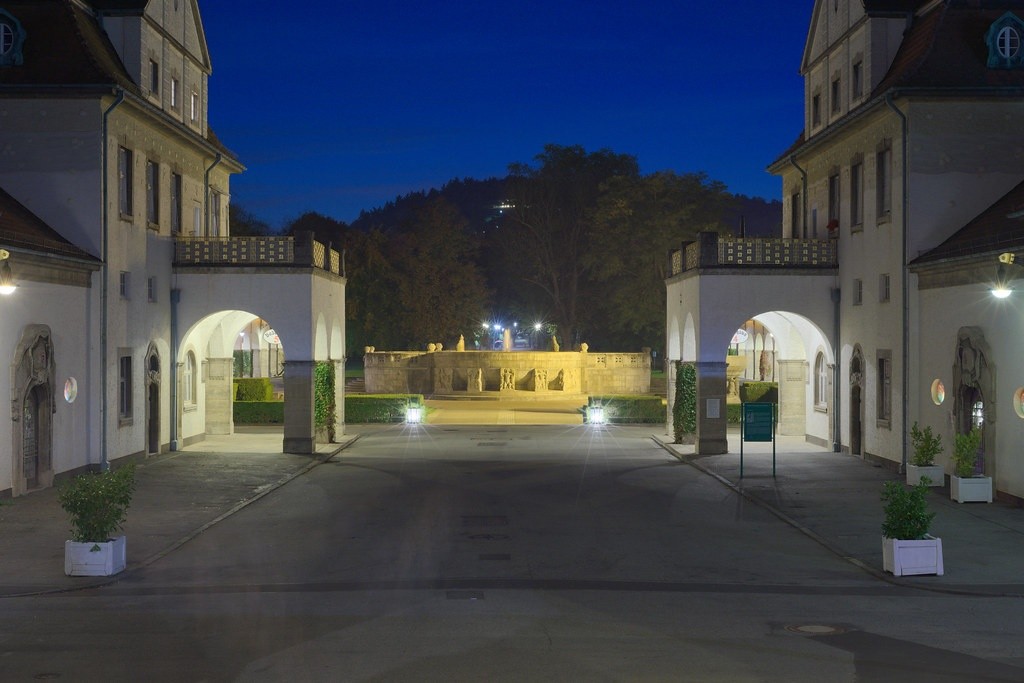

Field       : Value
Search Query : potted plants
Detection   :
[906,420,945,487]
[56,457,141,577]
[950,422,993,504]
[878,475,944,577]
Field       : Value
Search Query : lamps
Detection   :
[590,407,605,424]
[407,408,422,424]
[0,248,21,294]
[987,253,1024,299]
